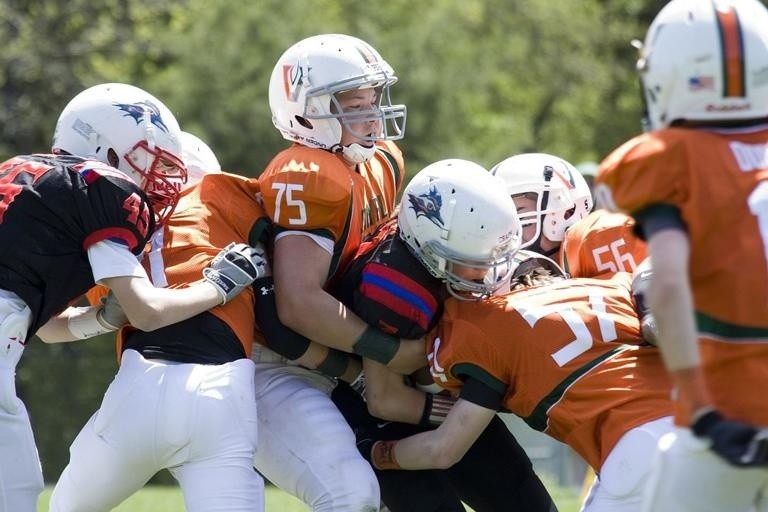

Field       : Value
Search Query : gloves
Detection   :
[687,403,768,468]
[98,289,130,330]
[352,368,384,473]
[200,241,272,306]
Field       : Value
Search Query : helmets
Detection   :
[398,158,523,278]
[50,82,224,231]
[635,1,768,131]
[490,152,594,241]
[266,32,409,166]
[484,249,567,298]
